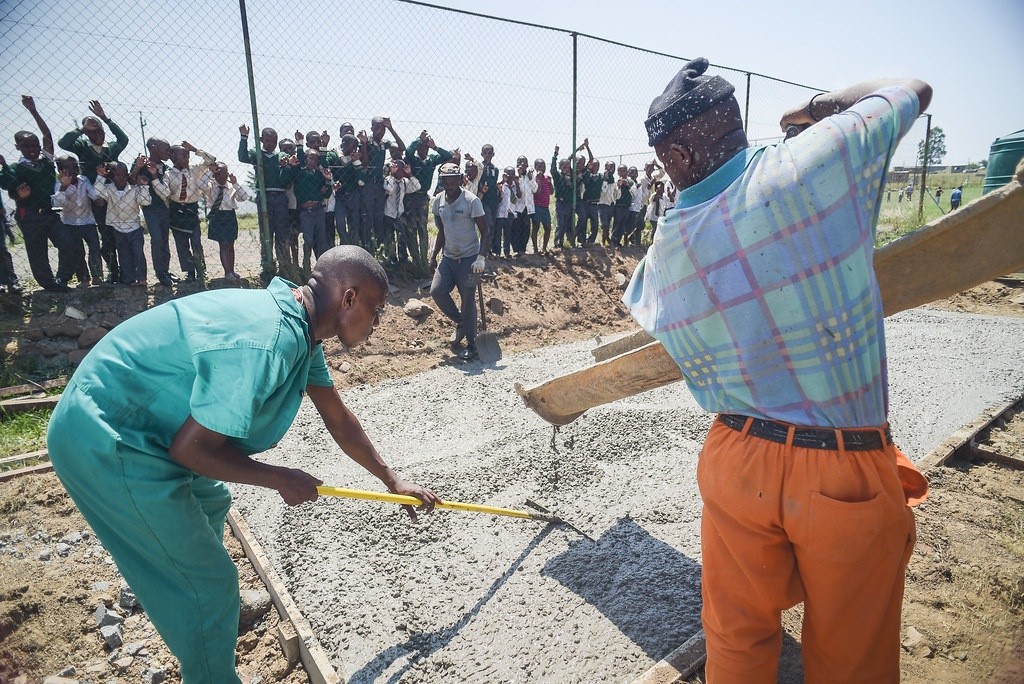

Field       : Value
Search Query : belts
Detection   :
[258,188,285,191]
[300,205,327,212]
[718,413,892,450]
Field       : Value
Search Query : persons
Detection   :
[886,189,891,202]
[898,188,904,203]
[935,187,943,204]
[237,116,681,273]
[0,95,249,292]
[427,163,490,360]
[905,184,913,201]
[948,186,962,213]
[620,57,933,684]
[45,245,445,684]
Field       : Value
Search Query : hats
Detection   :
[340,123,354,138]
[646,58,734,144]
[395,160,407,168]
[438,163,463,178]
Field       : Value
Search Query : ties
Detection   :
[180,175,187,202]
[206,186,226,221]
[394,181,402,217]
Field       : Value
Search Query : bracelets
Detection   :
[809,93,824,123]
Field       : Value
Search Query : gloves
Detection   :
[471,255,485,274]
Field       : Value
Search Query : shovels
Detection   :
[471,265,502,362]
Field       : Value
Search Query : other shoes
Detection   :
[450,317,464,343]
[0,268,241,294]
[461,345,478,360]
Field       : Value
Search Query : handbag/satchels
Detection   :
[168,200,199,235]
